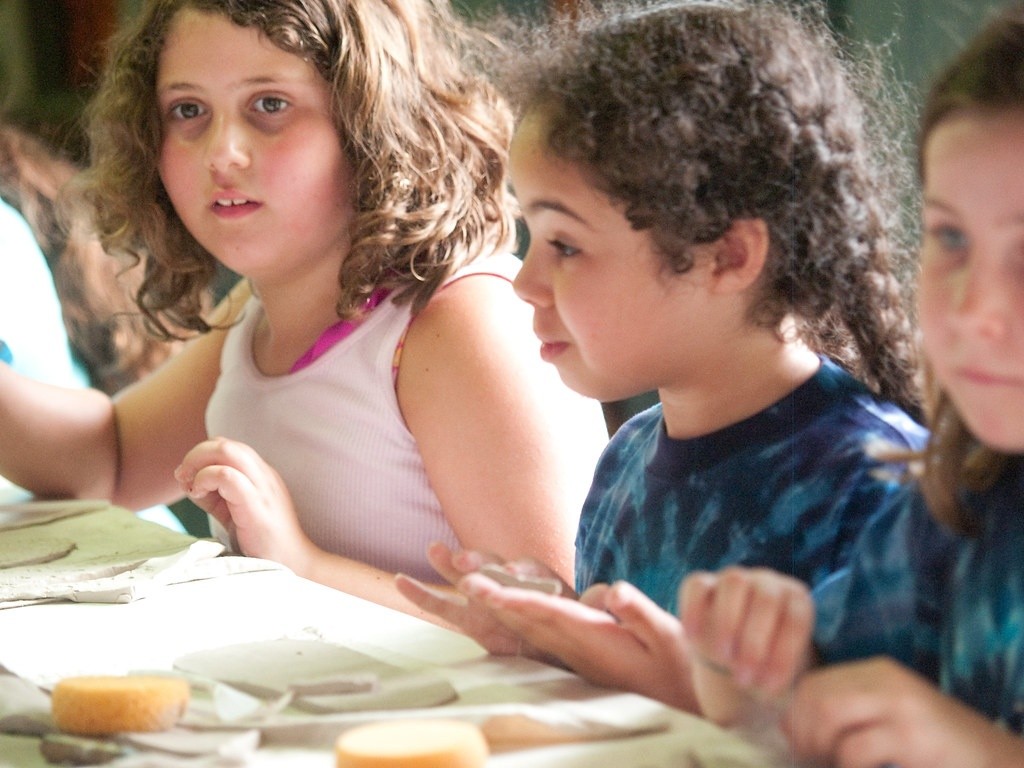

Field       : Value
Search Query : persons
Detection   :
[0,2,610,634]
[676,4,1023,768]
[395,0,931,717]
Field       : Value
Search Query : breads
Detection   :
[50,672,191,733]
[335,717,490,768]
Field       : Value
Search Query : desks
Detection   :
[0,478,786,768]
